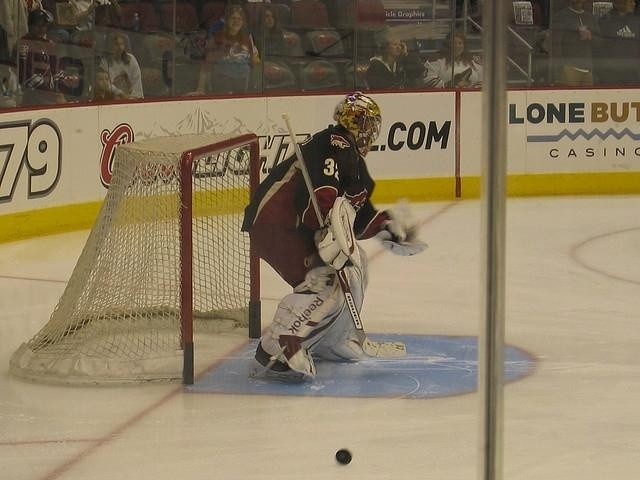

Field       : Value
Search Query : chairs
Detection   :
[17,0,543,95]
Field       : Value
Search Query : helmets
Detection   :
[333,91,382,157]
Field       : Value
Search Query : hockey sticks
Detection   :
[282,112,406,358]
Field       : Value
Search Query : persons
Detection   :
[0,1,145,108]
[365,25,425,91]
[183,4,303,96]
[531,0,640,89]
[422,31,488,88]
[241,94,427,376]
